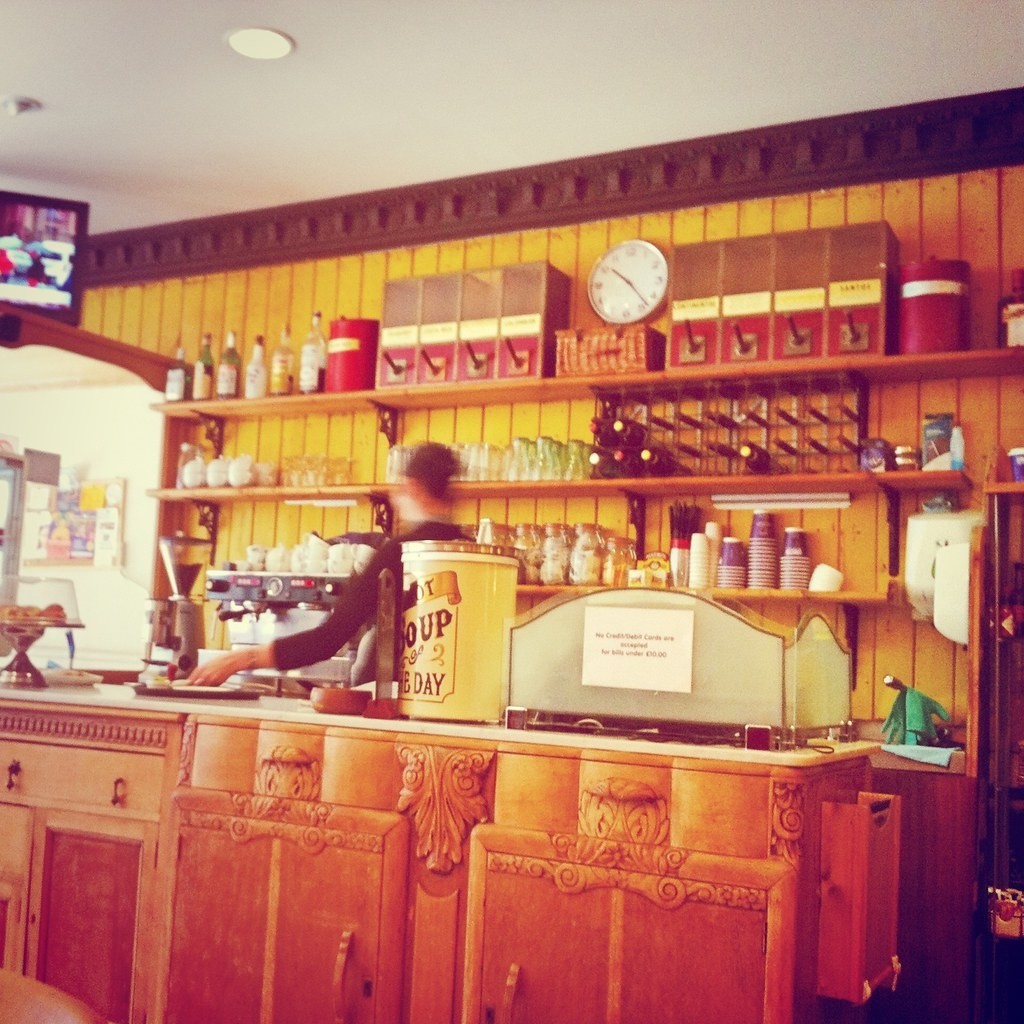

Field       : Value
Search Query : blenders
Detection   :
[158,534,210,678]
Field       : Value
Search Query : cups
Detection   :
[384,436,588,484]
[689,508,811,592]
[175,440,348,486]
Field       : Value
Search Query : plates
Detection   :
[172,686,235,692]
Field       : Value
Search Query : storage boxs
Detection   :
[374,263,571,381]
[665,221,899,370]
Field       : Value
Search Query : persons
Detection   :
[187,442,477,687]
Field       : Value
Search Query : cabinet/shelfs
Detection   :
[1,726,171,1024]
[160,777,899,1024]
[145,330,1024,612]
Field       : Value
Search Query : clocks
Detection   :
[586,239,670,327]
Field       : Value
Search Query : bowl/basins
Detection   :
[311,686,372,716]
[247,532,378,575]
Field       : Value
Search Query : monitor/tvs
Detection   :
[0,191,90,325]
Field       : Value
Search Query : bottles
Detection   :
[741,443,783,476]
[165,346,185,402]
[269,322,294,397]
[192,331,212,401]
[215,330,242,401]
[640,448,693,478]
[589,451,617,477]
[591,421,619,449]
[613,419,664,450]
[245,333,270,400]
[613,448,644,477]
[297,311,330,394]
[475,517,636,590]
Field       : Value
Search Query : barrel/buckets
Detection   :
[399,541,520,725]
